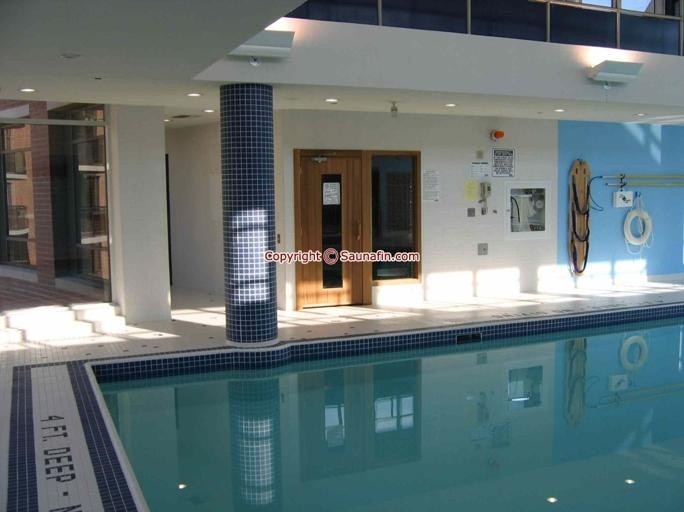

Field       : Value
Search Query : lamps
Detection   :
[226,31,294,67]
[586,58,644,91]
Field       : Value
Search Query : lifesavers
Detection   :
[623,209,651,244]
[621,337,647,372]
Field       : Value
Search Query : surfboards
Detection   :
[568,337,587,425]
[568,160,591,276]
